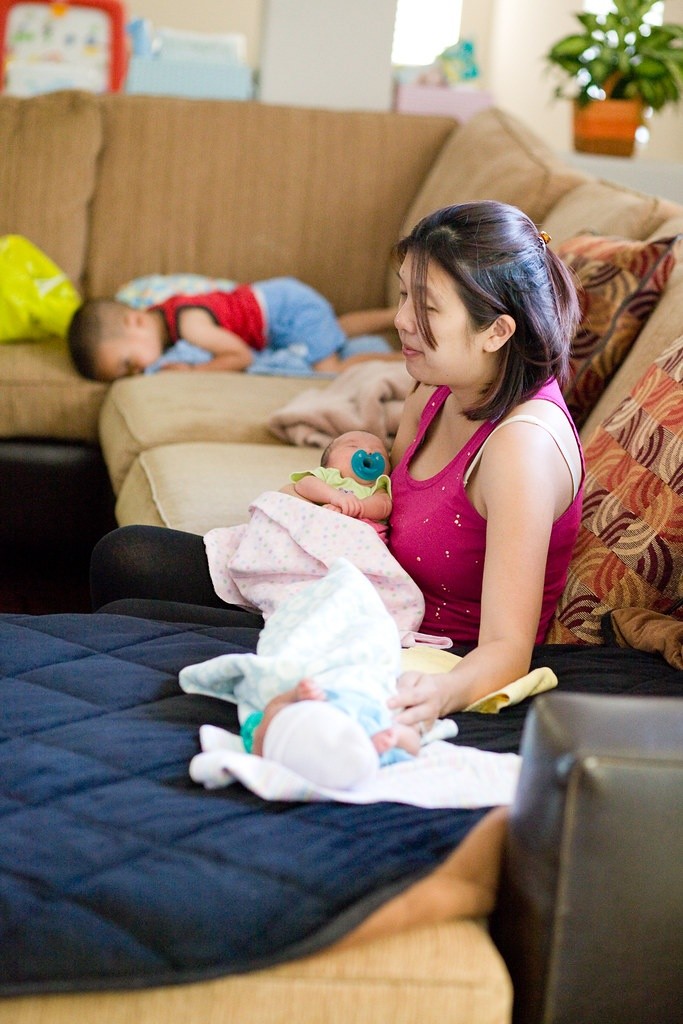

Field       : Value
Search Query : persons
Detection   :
[68,276,408,382]
[179,556,420,791]
[202,429,425,635]
[90,202,586,735]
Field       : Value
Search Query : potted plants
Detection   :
[548,0,683,157]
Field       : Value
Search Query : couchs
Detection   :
[0,91,682,1024]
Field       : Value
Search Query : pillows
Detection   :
[543,344,681,662]
[539,233,683,429]
